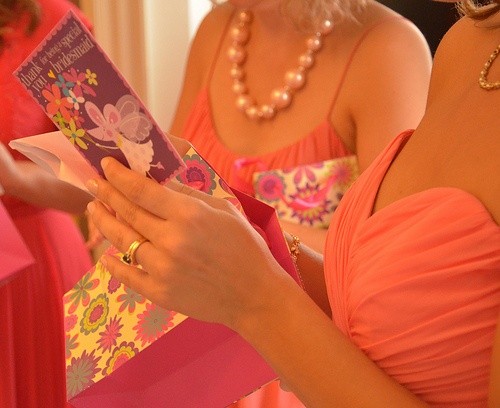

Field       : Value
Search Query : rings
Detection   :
[120,237,149,268]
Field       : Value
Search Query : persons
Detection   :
[168,0,433,408]
[0,0,94,408]
[86,0,499,408]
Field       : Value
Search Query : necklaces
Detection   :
[225,2,335,121]
[479,45,500,92]
